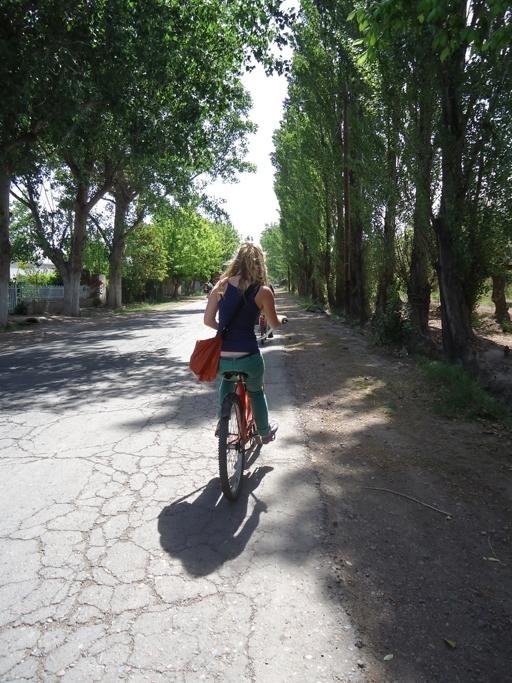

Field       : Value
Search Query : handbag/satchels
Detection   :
[189,334,223,383]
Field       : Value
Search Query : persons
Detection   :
[202,241,288,444]
[267,282,274,338]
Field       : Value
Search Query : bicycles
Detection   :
[212,316,288,502]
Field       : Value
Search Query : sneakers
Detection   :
[262,423,278,444]
[215,422,220,438]
